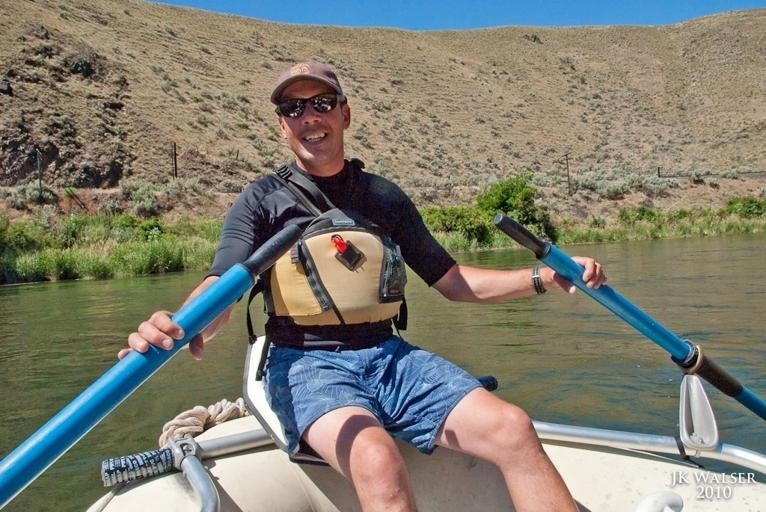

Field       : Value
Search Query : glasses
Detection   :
[275,93,345,118]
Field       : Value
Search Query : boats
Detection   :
[84,412,766,512]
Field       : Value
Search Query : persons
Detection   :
[117,62,609,512]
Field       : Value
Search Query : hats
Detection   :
[271,62,343,106]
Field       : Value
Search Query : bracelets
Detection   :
[530,267,548,295]
